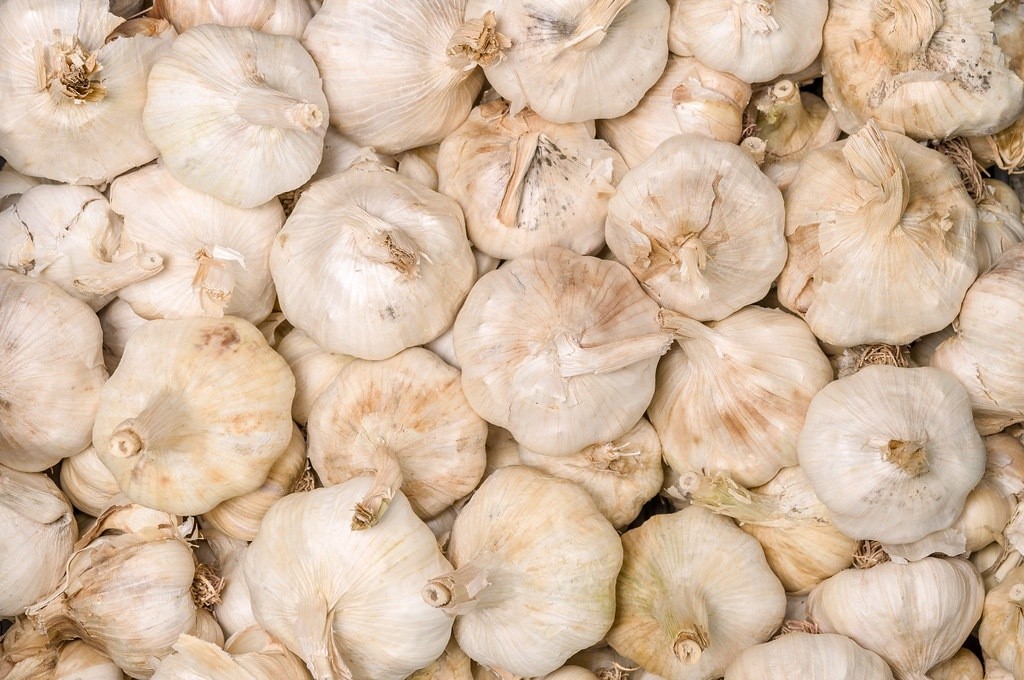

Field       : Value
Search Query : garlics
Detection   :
[0,0,1024,680]
